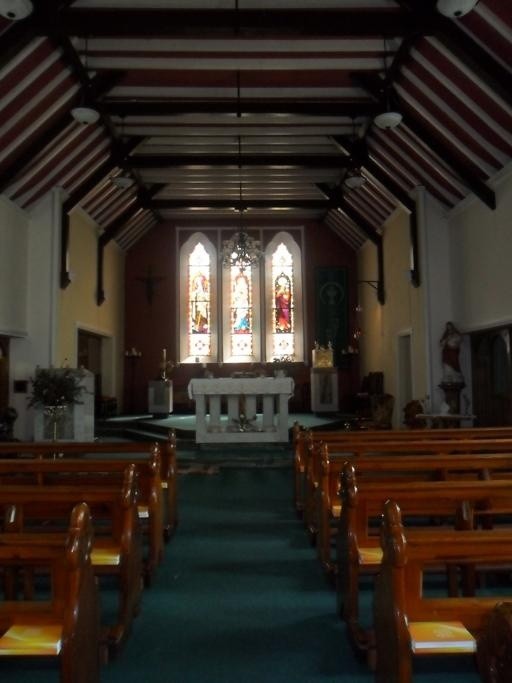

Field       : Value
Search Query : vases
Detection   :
[43,398,69,461]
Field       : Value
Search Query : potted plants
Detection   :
[271,354,297,378]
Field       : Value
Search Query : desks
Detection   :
[191,378,292,443]
[149,380,173,419]
[310,367,340,416]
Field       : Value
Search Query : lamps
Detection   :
[110,110,136,190]
[345,107,366,191]
[351,302,362,315]
[69,37,102,126]
[0,0,34,20]
[219,137,266,274]
[372,30,403,131]
[351,328,361,345]
[436,0,479,20]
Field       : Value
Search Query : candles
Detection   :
[162,346,167,372]
[342,346,361,354]
[125,348,144,357]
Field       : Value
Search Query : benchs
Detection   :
[0,427,177,683]
[292,420,512,683]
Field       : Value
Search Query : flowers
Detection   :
[25,357,96,417]
